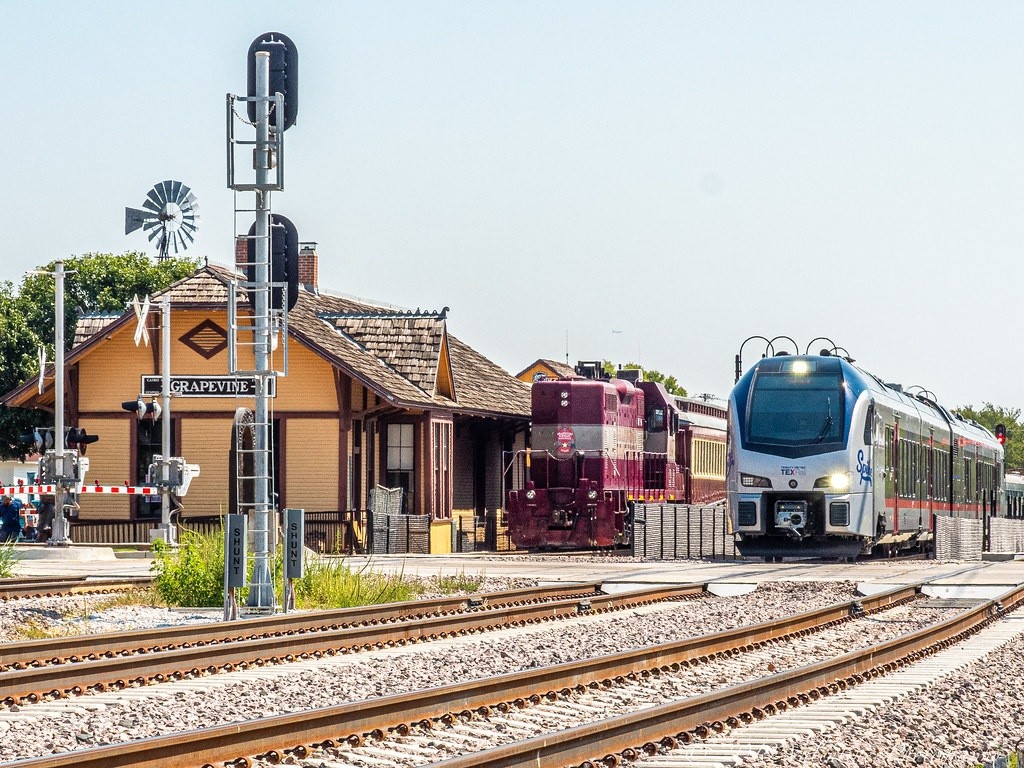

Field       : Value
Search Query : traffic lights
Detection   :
[65,426,85,449]
[20,432,44,454]
[77,427,98,455]
[994,423,1006,445]
[144,399,163,422]
[121,397,146,419]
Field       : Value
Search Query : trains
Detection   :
[726,336,1024,563]
[501,359,727,554]
